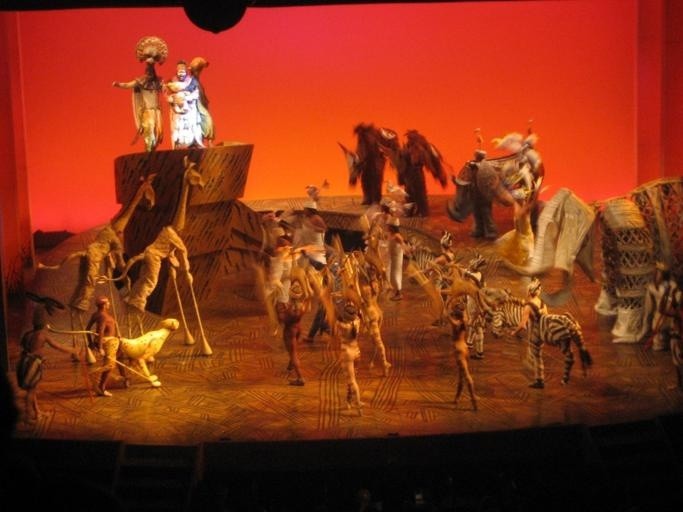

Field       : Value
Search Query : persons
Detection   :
[15,308,81,424]
[508,156,533,236]
[397,130,433,218]
[110,46,169,152]
[351,121,384,206]
[83,294,130,396]
[279,180,479,417]
[519,141,545,231]
[164,61,206,149]
[508,278,547,390]
[466,148,497,239]
[644,263,682,368]
